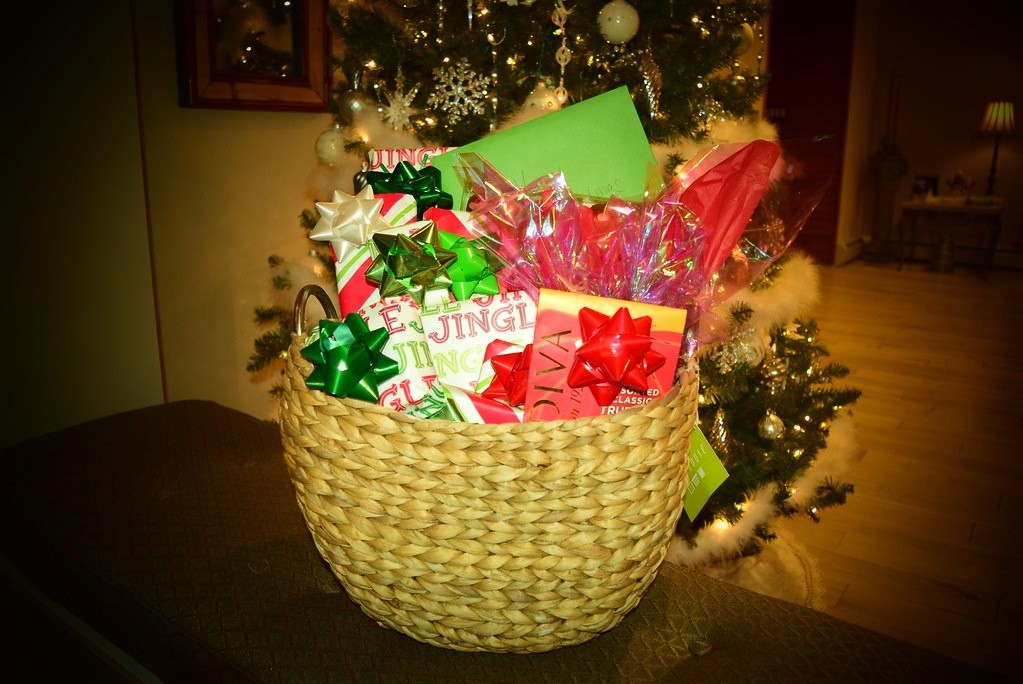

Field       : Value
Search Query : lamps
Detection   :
[975,102,1019,198]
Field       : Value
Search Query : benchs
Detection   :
[0,398,1023,684]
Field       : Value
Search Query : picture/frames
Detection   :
[176,0,334,113]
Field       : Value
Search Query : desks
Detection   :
[881,199,1010,276]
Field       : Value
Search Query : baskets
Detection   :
[269,286,711,653]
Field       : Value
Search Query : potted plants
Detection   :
[866,135,910,182]
